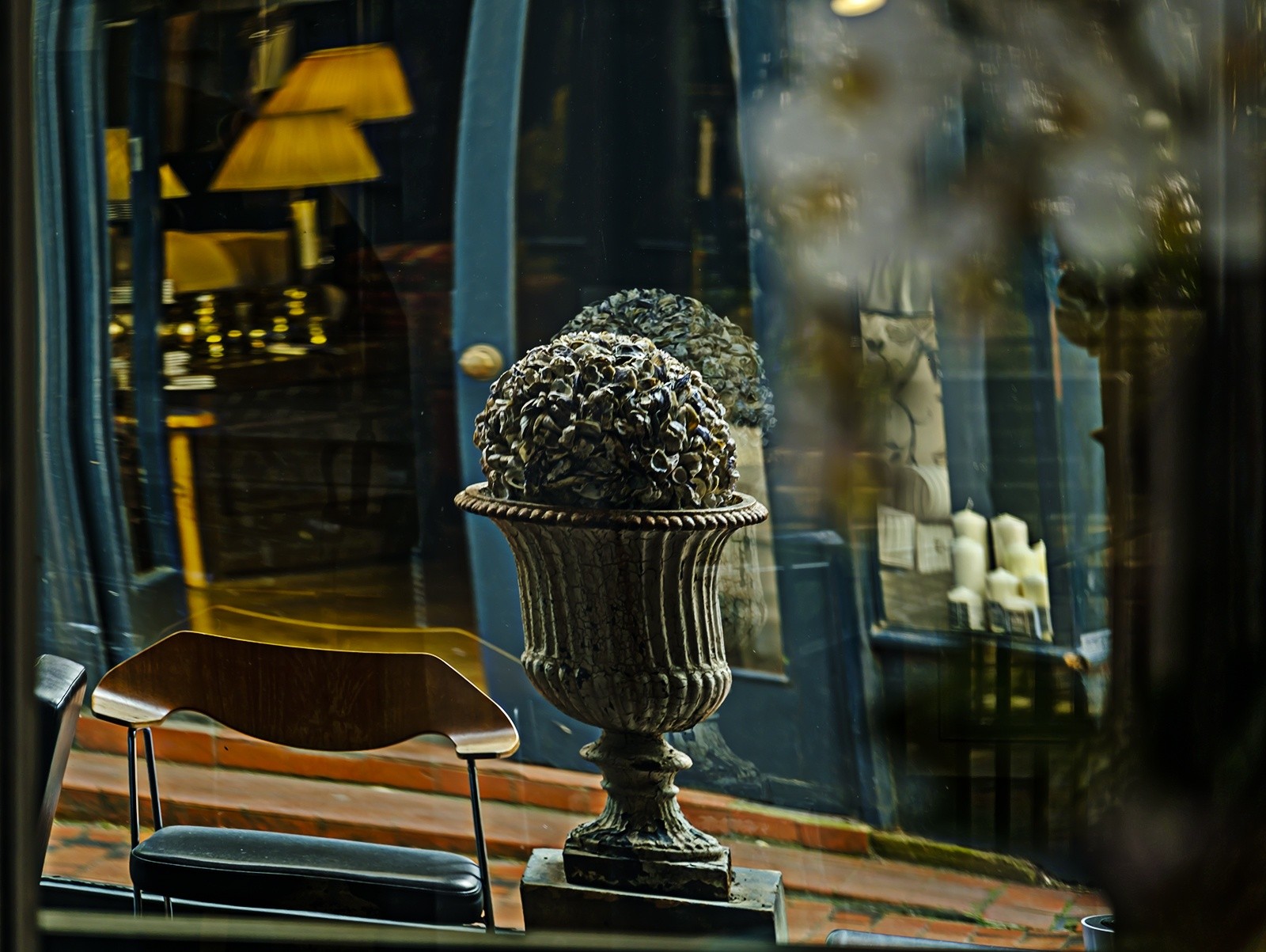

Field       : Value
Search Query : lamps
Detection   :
[269,43,411,292]
[212,115,379,356]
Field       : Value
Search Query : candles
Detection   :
[945,498,1051,664]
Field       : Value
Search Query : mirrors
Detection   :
[511,0,800,690]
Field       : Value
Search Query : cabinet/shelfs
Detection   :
[116,320,416,578]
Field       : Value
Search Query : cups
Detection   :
[1081,915,1113,952]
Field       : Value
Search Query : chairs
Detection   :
[90,630,520,939]
